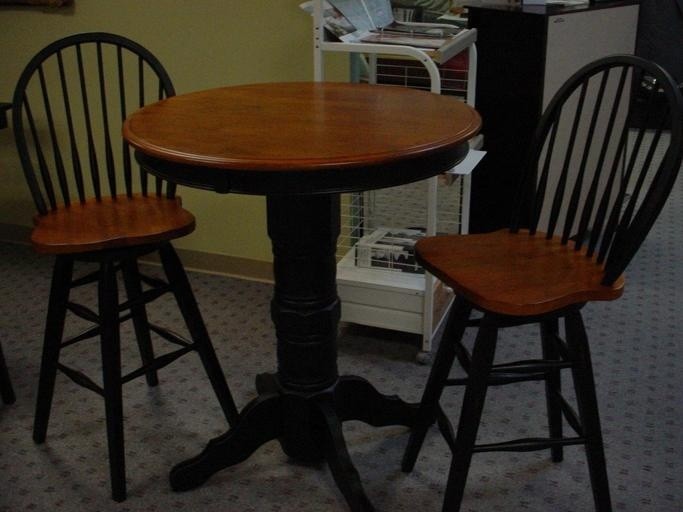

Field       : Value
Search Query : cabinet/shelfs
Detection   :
[309,2,477,360]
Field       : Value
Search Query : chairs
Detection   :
[402,36,682,508]
[10,35,252,495]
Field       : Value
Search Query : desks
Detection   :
[117,79,434,503]
